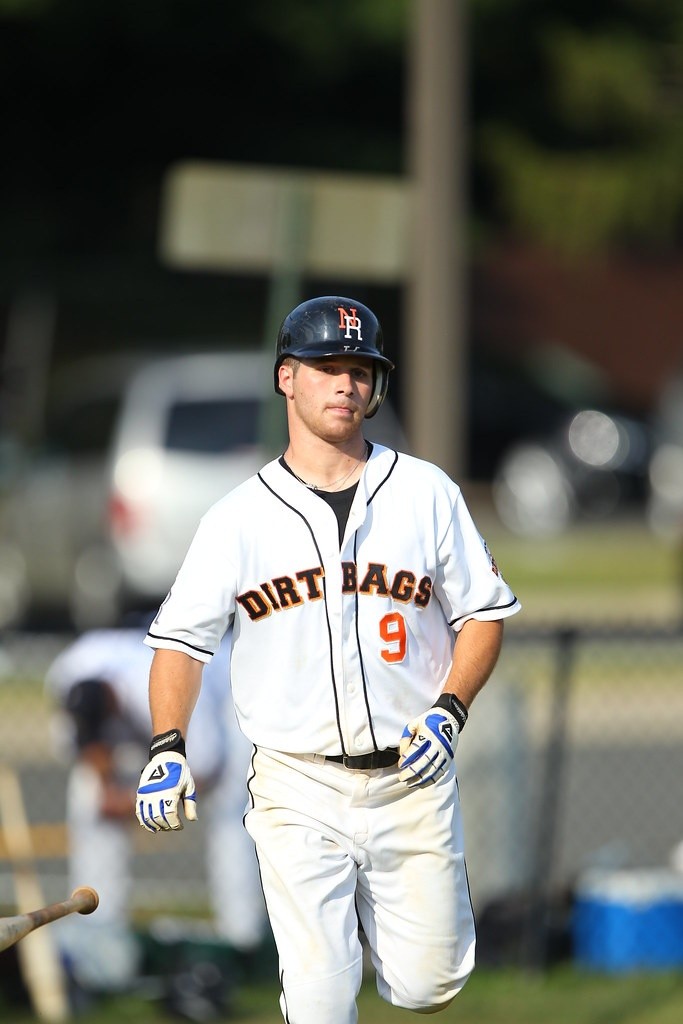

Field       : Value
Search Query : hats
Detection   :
[65,677,120,744]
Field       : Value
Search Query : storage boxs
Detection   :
[567,865,683,971]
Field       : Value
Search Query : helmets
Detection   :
[274,296,395,418]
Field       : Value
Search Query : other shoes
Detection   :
[230,949,274,989]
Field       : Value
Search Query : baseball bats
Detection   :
[1,887,99,951]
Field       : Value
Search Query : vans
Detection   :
[108,352,417,620]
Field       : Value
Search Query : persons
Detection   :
[62,730,140,988]
[47,627,274,991]
[132,292,524,1023]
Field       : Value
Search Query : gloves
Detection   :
[134,728,200,834]
[397,692,469,789]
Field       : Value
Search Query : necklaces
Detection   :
[281,442,368,490]
